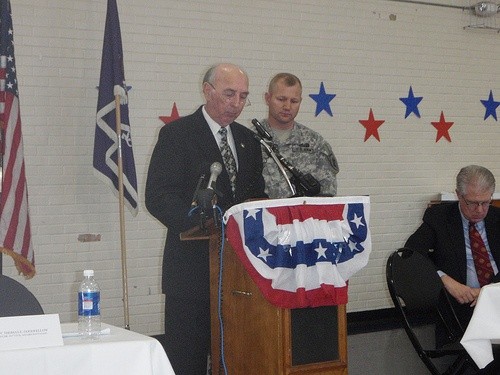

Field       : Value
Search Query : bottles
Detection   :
[78,270,101,339]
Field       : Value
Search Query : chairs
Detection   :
[386,248,500,375]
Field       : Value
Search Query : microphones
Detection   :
[251,119,272,140]
[191,161,222,209]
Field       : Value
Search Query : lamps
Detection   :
[475,2,500,16]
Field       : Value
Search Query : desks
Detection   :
[0,322,175,375]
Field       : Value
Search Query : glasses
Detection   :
[462,195,493,208]
[218,126,237,199]
[203,82,251,107]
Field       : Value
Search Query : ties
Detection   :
[468,222,495,288]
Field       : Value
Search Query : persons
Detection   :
[402,165,500,375]
[246,72,339,200]
[144,63,267,375]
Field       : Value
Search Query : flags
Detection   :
[0,0,36,279]
[92,0,140,217]
